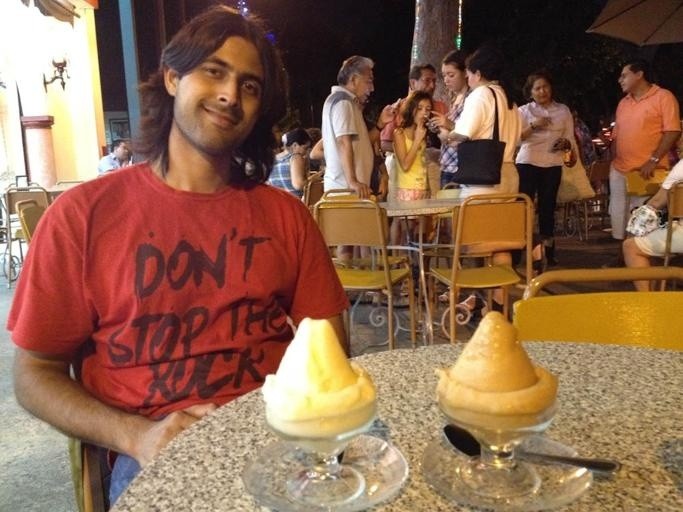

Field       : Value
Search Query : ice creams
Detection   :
[261,316,378,437]
[433,312,559,430]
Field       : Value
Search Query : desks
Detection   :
[108,333,683,508]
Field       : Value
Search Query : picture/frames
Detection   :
[107,118,129,142]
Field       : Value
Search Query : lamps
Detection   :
[40,52,74,91]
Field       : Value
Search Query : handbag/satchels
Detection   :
[451,139,506,184]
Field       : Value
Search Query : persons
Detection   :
[96,140,129,177]
[6,4,353,512]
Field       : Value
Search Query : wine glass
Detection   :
[239,401,408,512]
[418,395,591,512]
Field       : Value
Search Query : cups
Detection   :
[422,116,440,134]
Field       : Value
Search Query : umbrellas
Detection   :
[585,0,683,47]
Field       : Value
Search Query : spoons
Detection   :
[443,425,620,475]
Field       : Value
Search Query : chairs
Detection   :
[303,147,682,355]
[1,174,90,289]
[60,422,115,512]
